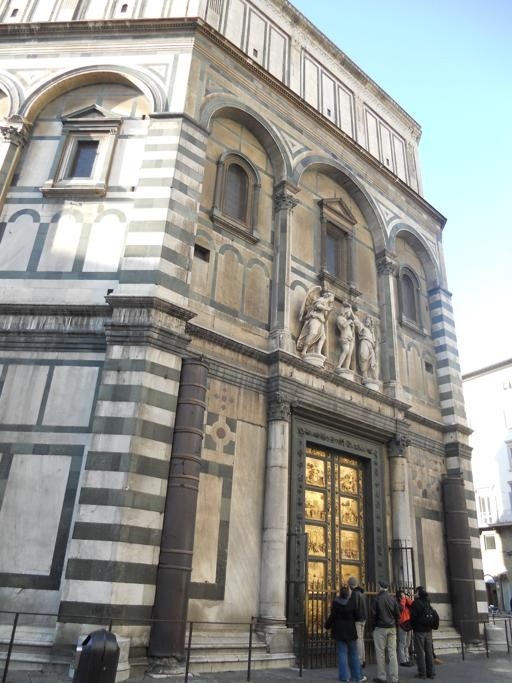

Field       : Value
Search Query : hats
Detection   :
[417,585,424,590]
[378,580,389,588]
[348,578,356,586]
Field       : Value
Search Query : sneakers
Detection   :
[414,672,437,680]
[359,676,368,683]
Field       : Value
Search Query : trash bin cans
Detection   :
[72,629,120,683]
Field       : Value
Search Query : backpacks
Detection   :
[422,607,439,631]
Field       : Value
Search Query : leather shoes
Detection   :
[399,661,415,667]
[373,678,387,683]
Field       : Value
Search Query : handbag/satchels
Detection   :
[400,620,413,632]
[331,608,358,642]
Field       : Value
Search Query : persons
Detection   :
[296,293,336,359]
[323,576,443,683]
[335,299,378,380]
